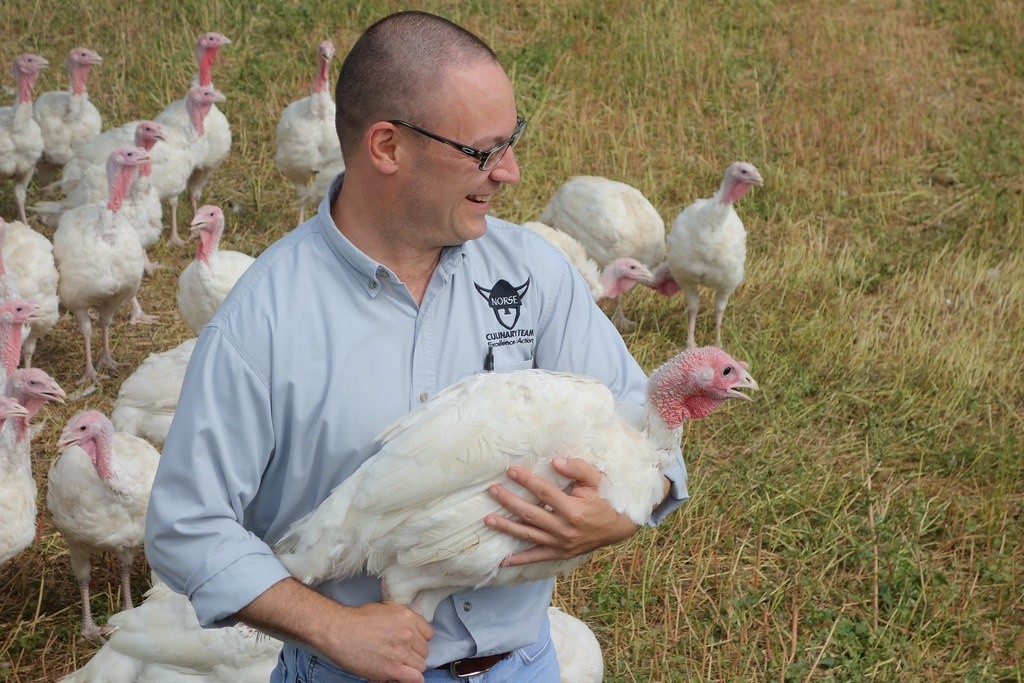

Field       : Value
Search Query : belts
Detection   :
[435,651,512,680]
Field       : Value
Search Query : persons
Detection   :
[143,11,691,683]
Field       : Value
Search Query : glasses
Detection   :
[386,115,527,172]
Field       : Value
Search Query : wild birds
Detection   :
[268,343,760,624]
[0,30,349,683]
[546,605,605,683]
[519,160,765,349]
